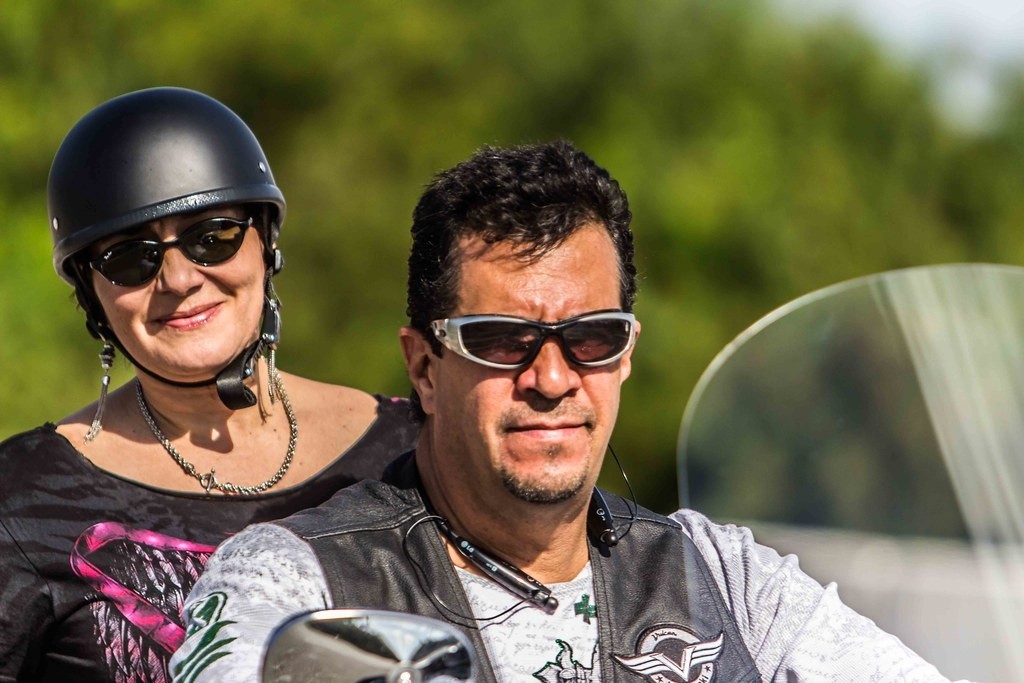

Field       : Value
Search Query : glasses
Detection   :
[79,207,261,288]
[410,309,636,370]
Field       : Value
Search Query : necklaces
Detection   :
[134,357,296,495]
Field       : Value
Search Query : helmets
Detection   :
[48,87,286,286]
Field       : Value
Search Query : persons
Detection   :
[169,139,951,683]
[0,87,419,683]
